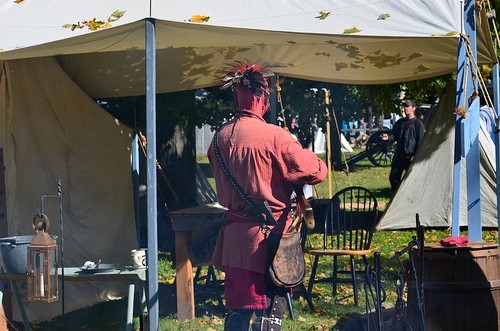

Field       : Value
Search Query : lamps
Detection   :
[25,214,59,304]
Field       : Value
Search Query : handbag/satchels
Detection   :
[264,230,306,288]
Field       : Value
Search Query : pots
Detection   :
[0,235,59,273]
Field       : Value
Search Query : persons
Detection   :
[380,100,425,202]
[206,57,328,331]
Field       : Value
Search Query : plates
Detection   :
[79,264,124,272]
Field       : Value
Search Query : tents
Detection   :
[375,79,499,230]
[0,0,496,321]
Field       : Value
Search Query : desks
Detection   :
[0,265,149,331]
[167,202,226,318]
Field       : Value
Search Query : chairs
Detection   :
[307,186,387,308]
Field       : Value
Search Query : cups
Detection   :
[131,250,147,269]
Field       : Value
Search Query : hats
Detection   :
[402,100,416,106]
